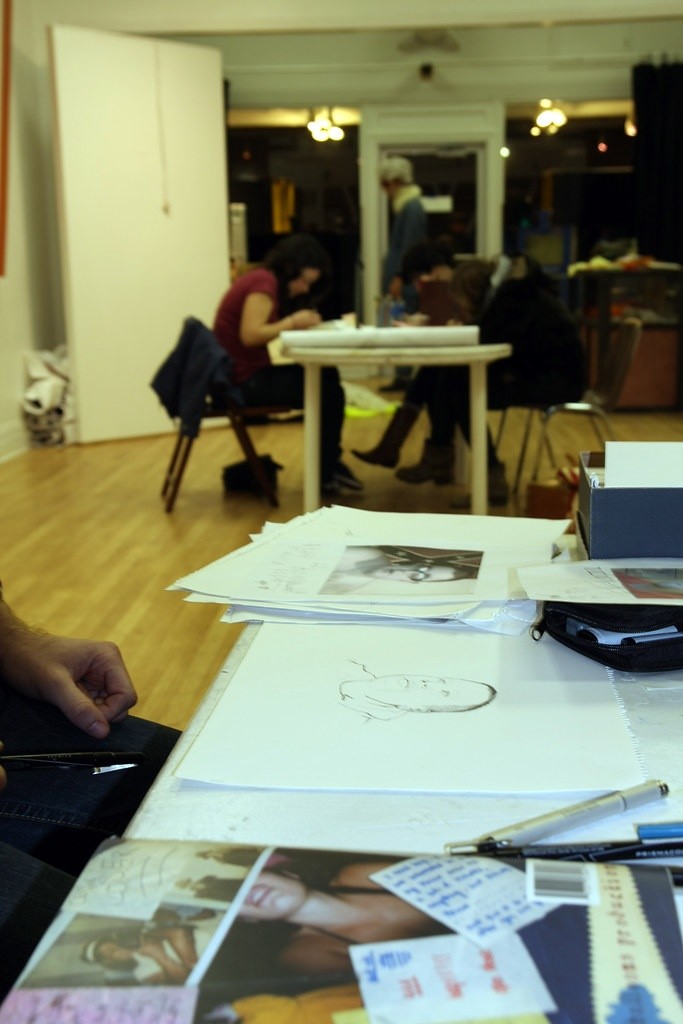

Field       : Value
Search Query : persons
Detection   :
[178,874,243,903]
[200,846,260,867]
[153,907,218,921]
[80,922,199,986]
[378,154,429,393]
[240,857,440,979]
[0,586,182,999]
[212,233,363,499]
[353,247,590,509]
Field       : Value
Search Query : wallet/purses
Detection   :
[532,600,683,673]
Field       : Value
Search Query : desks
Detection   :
[282,345,512,514]
[578,310,681,409]
[0,618,683,1024]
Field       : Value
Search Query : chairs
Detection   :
[159,316,279,515]
[495,314,641,493]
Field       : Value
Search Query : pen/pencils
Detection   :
[450,779,669,855]
[445,833,683,859]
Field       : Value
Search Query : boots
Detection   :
[351,407,418,468]
[394,438,455,486]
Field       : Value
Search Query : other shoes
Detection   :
[328,460,364,490]
[448,463,509,508]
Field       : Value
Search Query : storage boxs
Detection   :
[577,451,683,560]
